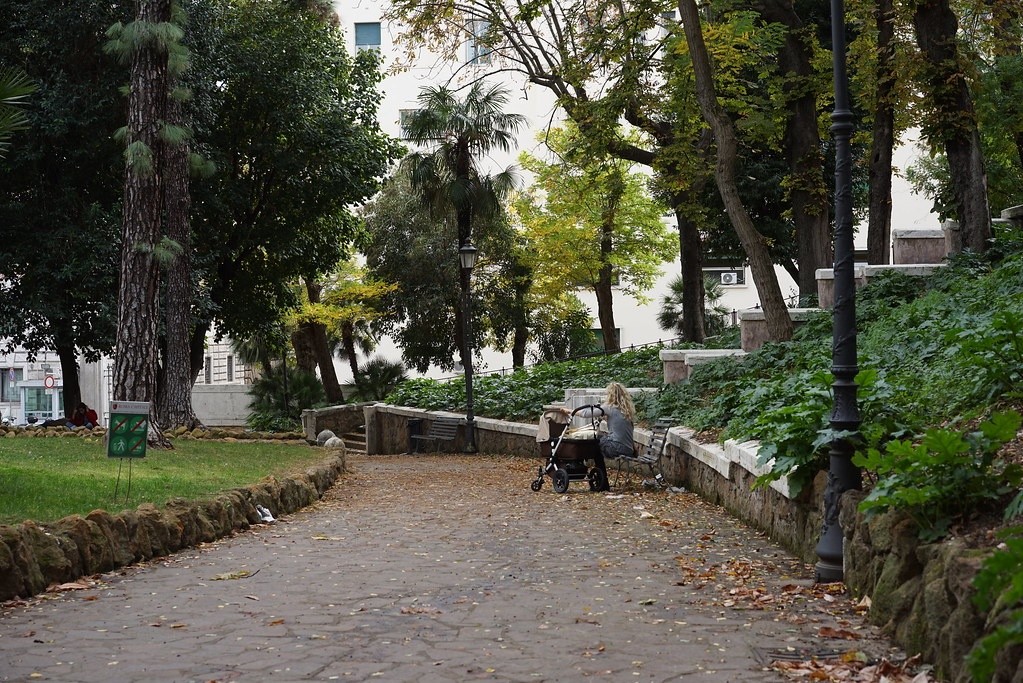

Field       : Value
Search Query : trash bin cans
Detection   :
[407,419,424,454]
[107,399,150,457]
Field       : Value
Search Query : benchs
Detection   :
[412,417,460,456]
[615,417,677,496]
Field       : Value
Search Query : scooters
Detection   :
[1,415,38,427]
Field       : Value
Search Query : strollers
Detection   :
[530,403,606,493]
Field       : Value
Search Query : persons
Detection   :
[65,402,100,430]
[561,382,636,460]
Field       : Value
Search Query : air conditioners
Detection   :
[721,273,738,284]
